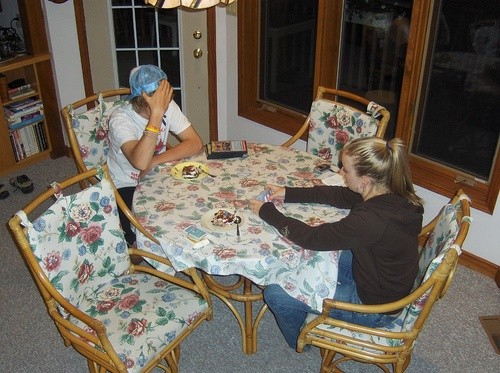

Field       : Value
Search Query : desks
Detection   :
[130,143,353,355]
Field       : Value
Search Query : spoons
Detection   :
[234,216,241,237]
[196,167,217,178]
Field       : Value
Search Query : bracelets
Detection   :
[143,124,161,140]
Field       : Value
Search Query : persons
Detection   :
[248,136,424,351]
[382,0,412,74]
[107,65,203,265]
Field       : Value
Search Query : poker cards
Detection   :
[258,188,276,204]
[184,225,207,242]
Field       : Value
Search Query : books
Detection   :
[7,78,36,100]
[4,96,49,162]
[205,140,248,160]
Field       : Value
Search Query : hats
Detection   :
[124,64,167,102]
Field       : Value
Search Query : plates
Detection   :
[171,162,210,181]
[200,208,244,232]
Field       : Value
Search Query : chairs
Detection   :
[60,87,141,266]
[7,163,213,373]
[297,190,474,373]
[280,87,391,168]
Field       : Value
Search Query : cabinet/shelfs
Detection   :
[0,0,67,180]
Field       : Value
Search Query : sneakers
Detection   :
[9,174,35,194]
[0,184,9,200]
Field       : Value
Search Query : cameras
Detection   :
[254,189,274,204]
